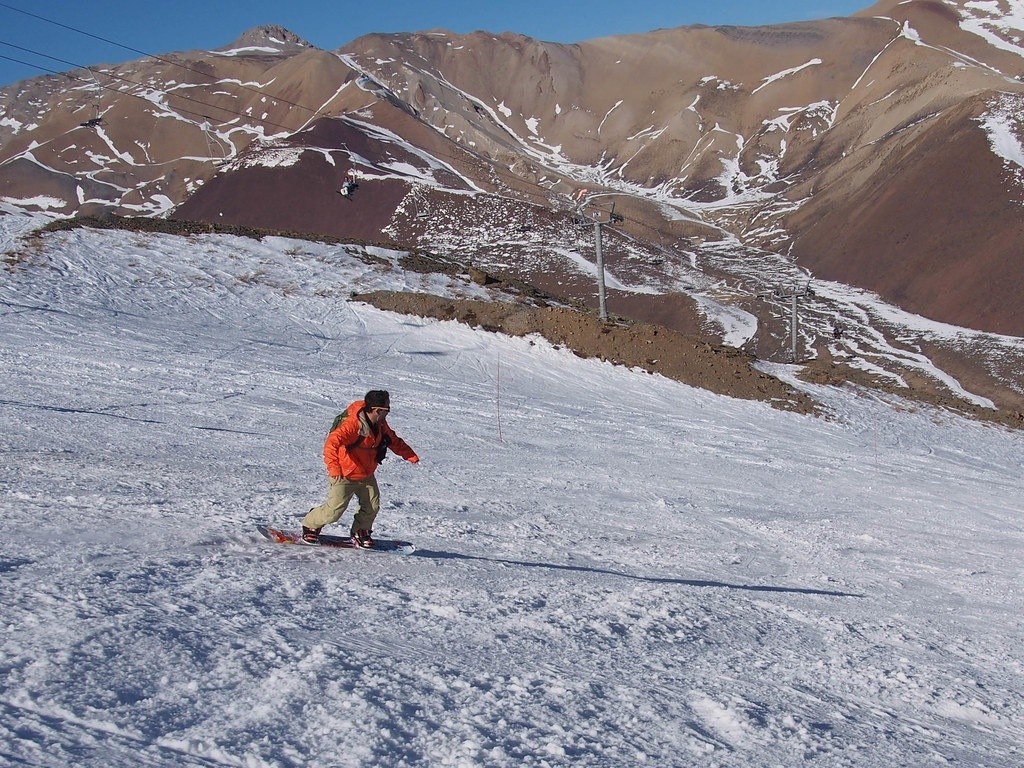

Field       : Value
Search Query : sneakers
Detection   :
[349,528,375,551]
[300,525,322,547]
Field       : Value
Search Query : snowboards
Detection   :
[255,524,417,556]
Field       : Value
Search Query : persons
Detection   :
[301,390,419,548]
[341,176,351,195]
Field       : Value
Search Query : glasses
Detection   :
[371,406,390,412]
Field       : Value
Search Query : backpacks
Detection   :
[329,408,385,452]
